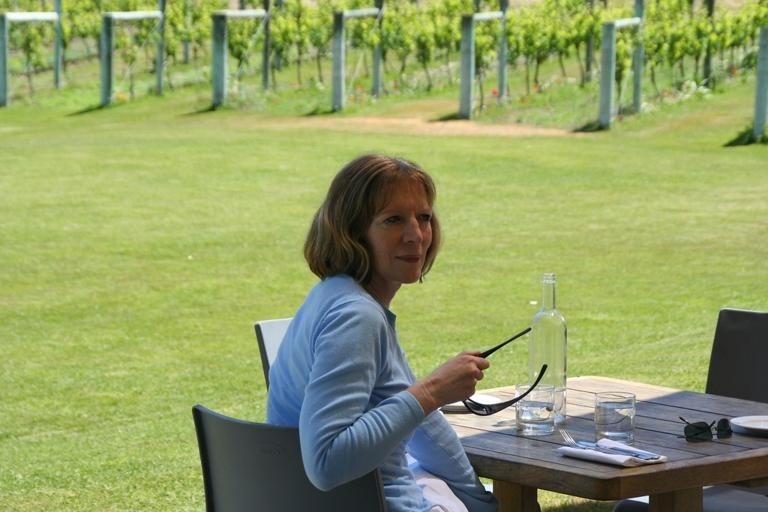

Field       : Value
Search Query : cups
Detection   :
[515,384,555,436]
[593,391,635,447]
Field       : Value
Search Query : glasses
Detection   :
[679,416,734,443]
[462,326,548,416]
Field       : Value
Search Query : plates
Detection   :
[730,415,768,432]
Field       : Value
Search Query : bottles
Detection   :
[529,273,567,423]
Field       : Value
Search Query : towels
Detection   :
[557,437,668,468]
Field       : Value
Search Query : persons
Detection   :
[264,148,542,512]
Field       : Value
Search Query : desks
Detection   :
[439,373,768,511]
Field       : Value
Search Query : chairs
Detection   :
[191,408,390,512]
[254,315,300,391]
[615,308,767,510]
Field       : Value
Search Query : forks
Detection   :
[559,429,652,460]
